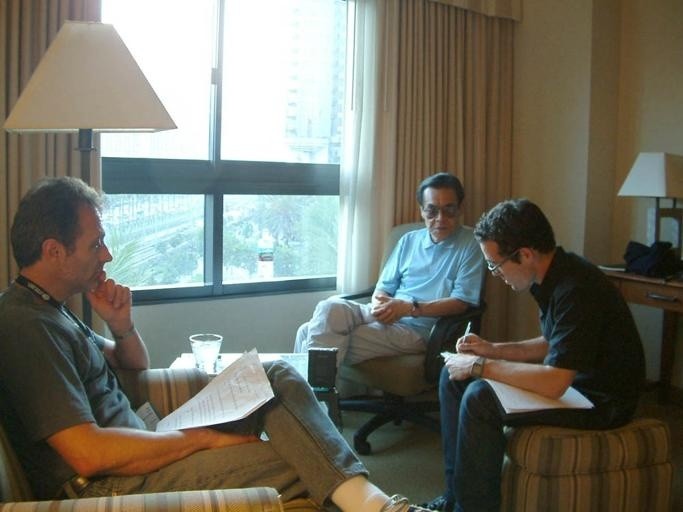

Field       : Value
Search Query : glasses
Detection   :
[421,207,456,218]
[487,249,519,274]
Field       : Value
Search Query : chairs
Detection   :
[326,223,489,455]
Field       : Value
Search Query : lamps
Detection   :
[2,19,177,328]
[616,151,682,259]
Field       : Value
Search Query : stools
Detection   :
[501,418,674,512]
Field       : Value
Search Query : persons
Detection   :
[0,175,438,512]
[410,200,646,512]
[293,173,488,397]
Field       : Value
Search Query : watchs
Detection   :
[411,297,422,319]
[469,356,487,378]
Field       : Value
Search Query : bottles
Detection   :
[256,228,276,281]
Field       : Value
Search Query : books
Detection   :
[438,350,595,415]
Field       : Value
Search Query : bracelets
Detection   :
[111,320,137,339]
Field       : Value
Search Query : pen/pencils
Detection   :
[462,321,472,343]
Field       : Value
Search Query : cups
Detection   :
[189,333,223,369]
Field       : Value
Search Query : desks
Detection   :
[601,266,683,400]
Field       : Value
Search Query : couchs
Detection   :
[1,367,282,511]
